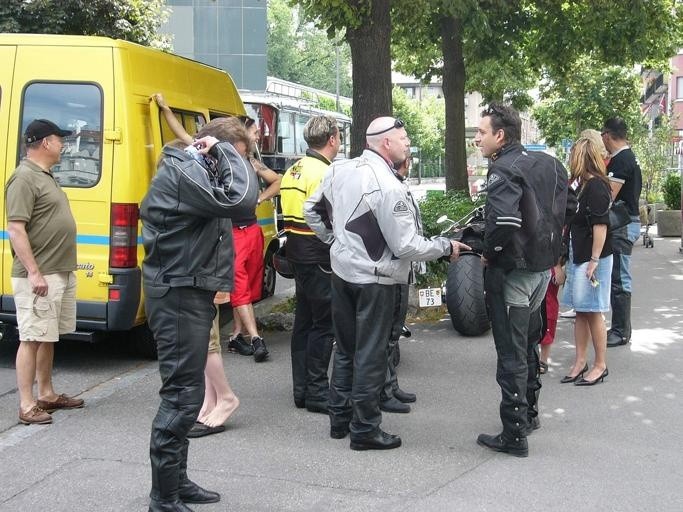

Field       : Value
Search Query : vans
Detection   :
[0,29,287,366]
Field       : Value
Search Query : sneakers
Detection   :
[250,335,269,362]
[37,394,84,412]
[227,332,254,355]
[17,406,53,424]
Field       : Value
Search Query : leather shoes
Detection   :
[148,498,195,512]
[293,391,416,448]
[477,433,528,456]
[177,484,220,503]
[608,333,629,346]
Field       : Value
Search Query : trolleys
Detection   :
[637,198,654,250]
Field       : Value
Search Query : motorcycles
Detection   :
[434,177,489,342]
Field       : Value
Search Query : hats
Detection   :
[25,119,72,143]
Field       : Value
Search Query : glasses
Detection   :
[486,108,496,115]
[365,117,405,135]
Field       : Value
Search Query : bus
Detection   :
[236,87,353,186]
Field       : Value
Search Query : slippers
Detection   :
[188,421,226,437]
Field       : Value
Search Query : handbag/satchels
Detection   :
[586,200,632,235]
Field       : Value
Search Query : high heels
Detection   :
[560,361,588,383]
[577,366,609,385]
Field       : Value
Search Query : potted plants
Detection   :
[624,96,682,237]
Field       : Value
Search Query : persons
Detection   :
[476,107,642,458]
[139,91,282,511]
[5,119,84,424]
[276,115,472,451]
